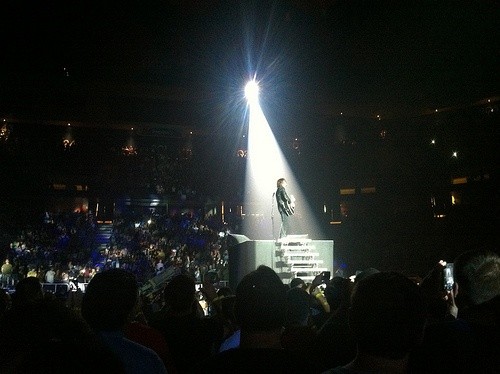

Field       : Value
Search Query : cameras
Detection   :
[324,271,330,280]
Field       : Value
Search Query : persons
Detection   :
[233,253,500,374]
[1,201,247,374]
[276,178,295,239]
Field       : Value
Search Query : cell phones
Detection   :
[443,263,455,293]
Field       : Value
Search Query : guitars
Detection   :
[283,194,296,216]
